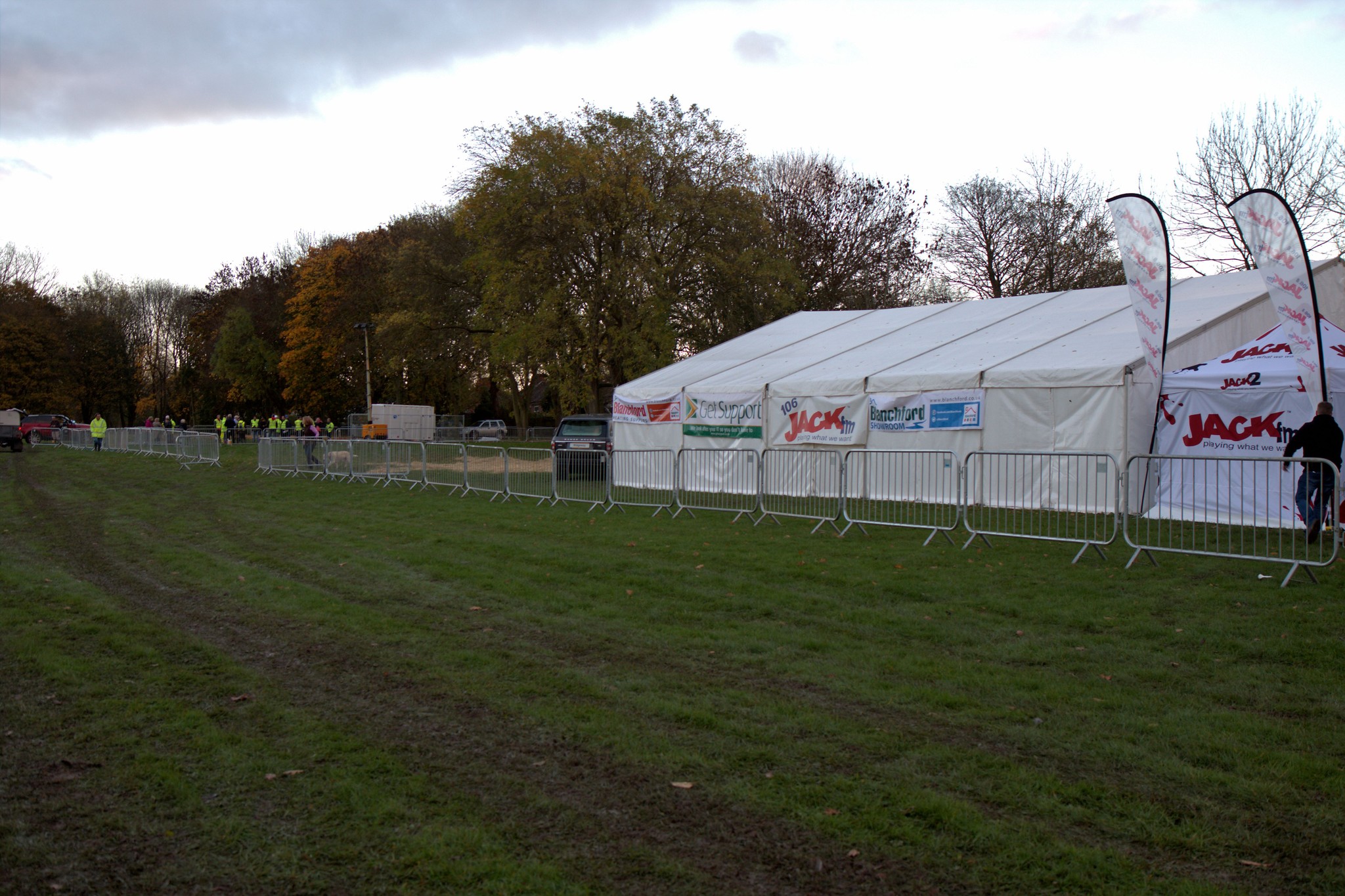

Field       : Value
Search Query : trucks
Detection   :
[0,410,23,454]
[347,414,388,440]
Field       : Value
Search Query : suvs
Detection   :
[20,415,92,445]
[461,419,508,440]
[551,413,616,480]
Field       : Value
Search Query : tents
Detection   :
[1147,310,1344,535]
[613,256,1345,535]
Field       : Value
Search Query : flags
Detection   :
[1105,192,1174,517]
[1227,189,1331,419]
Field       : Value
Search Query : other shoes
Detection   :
[1307,518,1321,544]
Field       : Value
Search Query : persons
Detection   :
[89,412,107,451]
[50,416,61,444]
[145,413,335,442]
[1280,401,1344,545]
[301,415,323,469]
